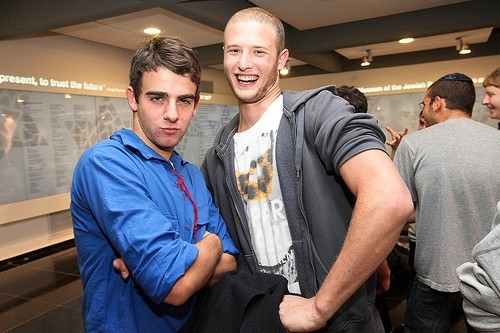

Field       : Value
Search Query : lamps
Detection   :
[361,49,372,66]
[456,37,471,55]
[280,60,290,75]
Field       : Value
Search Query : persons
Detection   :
[314,75,450,333]
[71,34,241,333]
[389,73,500,333]
[481,67,500,131]
[456,200,500,333]
[195,6,412,333]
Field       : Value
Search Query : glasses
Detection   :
[419,96,445,110]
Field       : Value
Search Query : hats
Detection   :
[438,72,473,83]
